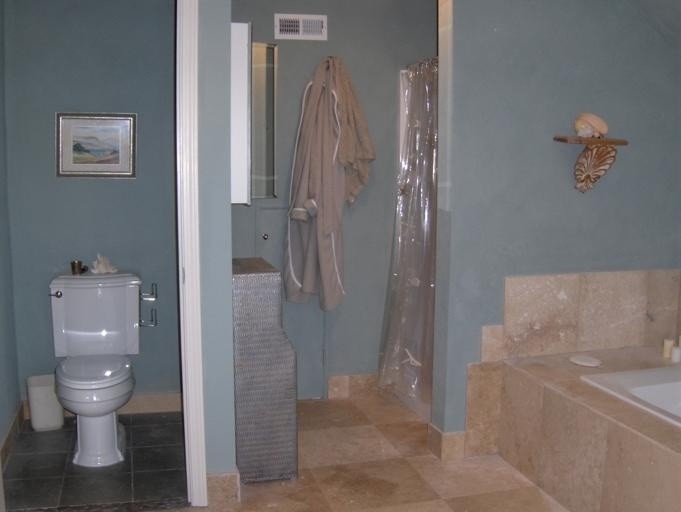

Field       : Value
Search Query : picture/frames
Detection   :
[54,110,137,178]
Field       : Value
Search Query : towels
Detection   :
[324,55,376,202]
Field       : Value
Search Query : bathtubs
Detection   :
[579,365,681,428]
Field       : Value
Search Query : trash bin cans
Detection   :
[26,374,64,432]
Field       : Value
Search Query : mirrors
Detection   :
[252,41,277,200]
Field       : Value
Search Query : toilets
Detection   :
[49,273,157,467]
